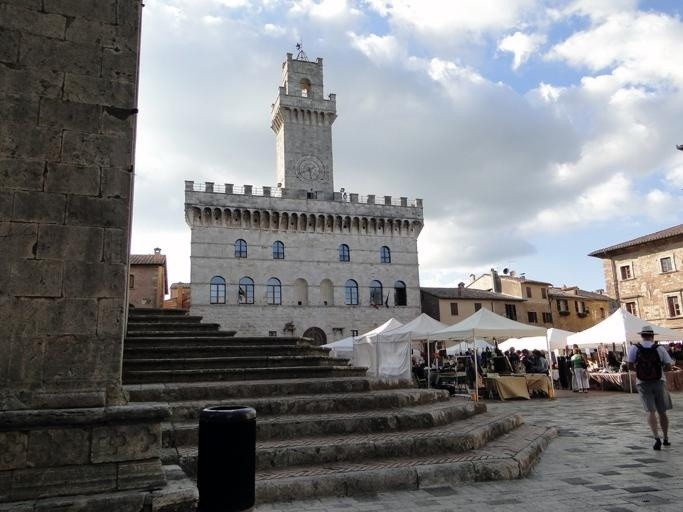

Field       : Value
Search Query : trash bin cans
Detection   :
[197,405,257,512]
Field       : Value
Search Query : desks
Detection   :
[483,367,682,401]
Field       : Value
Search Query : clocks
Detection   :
[294,155,326,186]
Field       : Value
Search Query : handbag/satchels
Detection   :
[580,357,589,369]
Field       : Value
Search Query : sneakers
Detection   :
[579,390,589,393]
[653,438,662,450]
[663,438,671,446]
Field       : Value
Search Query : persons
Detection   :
[339,187,346,201]
[623,326,675,450]
[405,340,683,394]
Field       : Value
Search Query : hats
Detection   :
[635,326,659,336]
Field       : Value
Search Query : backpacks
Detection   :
[634,342,663,381]
